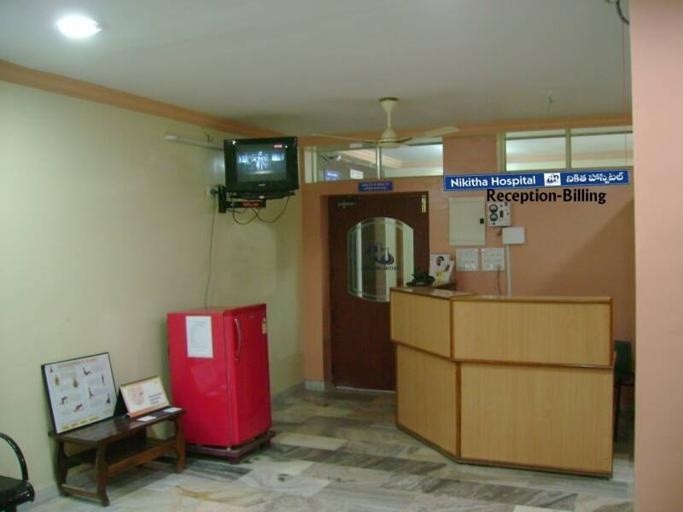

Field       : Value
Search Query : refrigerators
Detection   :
[167,303,271,447]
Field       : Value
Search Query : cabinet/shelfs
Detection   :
[166,304,277,464]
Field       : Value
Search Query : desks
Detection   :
[46,406,186,506]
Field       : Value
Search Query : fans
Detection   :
[310,97,460,149]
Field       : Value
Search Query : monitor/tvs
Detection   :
[224,136,298,196]
